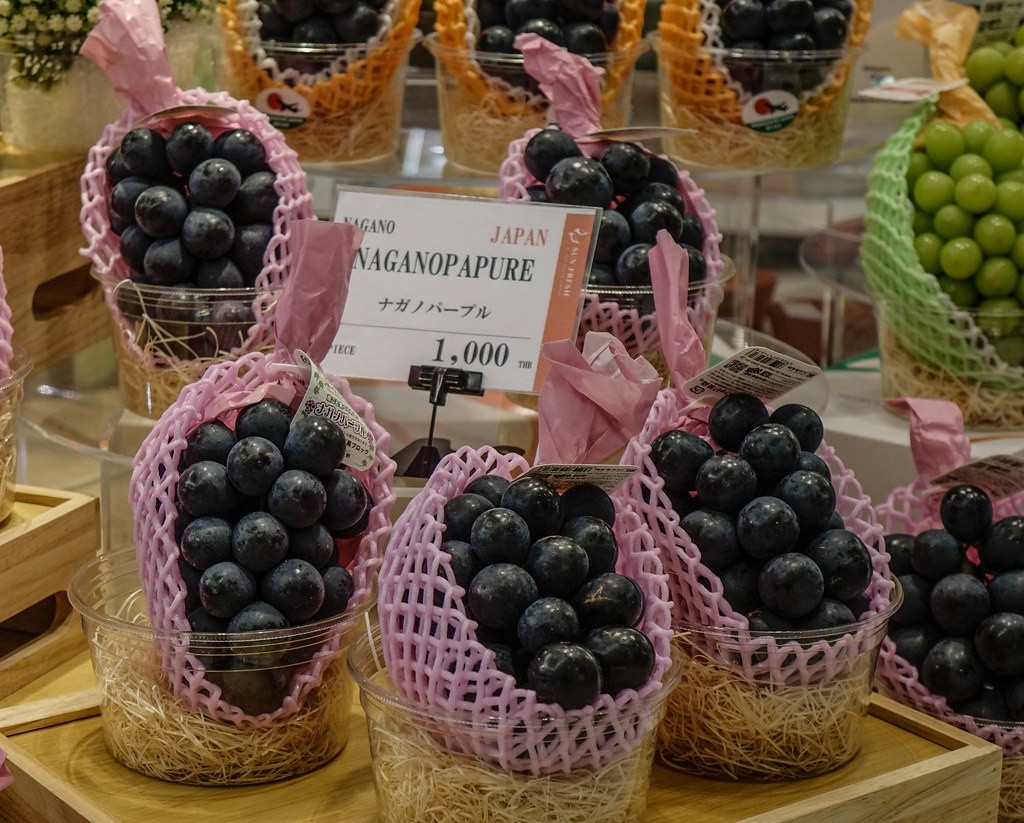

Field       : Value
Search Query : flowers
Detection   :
[0,0,102,92]
[159,0,220,33]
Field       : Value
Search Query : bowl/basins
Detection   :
[877,300,1023,433]
[344,606,682,823]
[641,32,866,173]
[572,251,735,389]
[68,543,381,790]
[423,32,650,177]
[0,343,34,523]
[657,568,904,785]
[214,27,423,166]
[870,675,1024,823]
[106,276,288,421]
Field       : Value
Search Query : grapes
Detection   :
[256,1,855,348]
[633,390,873,675]
[155,399,376,716]
[868,484,1024,723]
[399,475,661,757]
[106,121,284,364]
[897,26,1024,375]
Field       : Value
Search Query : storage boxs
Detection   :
[0,27,1024,823]
